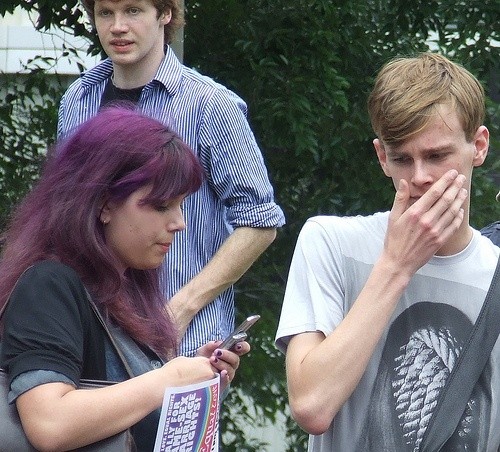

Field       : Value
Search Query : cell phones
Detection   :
[211,316,260,357]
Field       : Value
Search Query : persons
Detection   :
[273,51,500,452]
[0,110,253,452]
[60,0,288,361]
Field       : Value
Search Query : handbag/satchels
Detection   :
[0,262,138,452]
